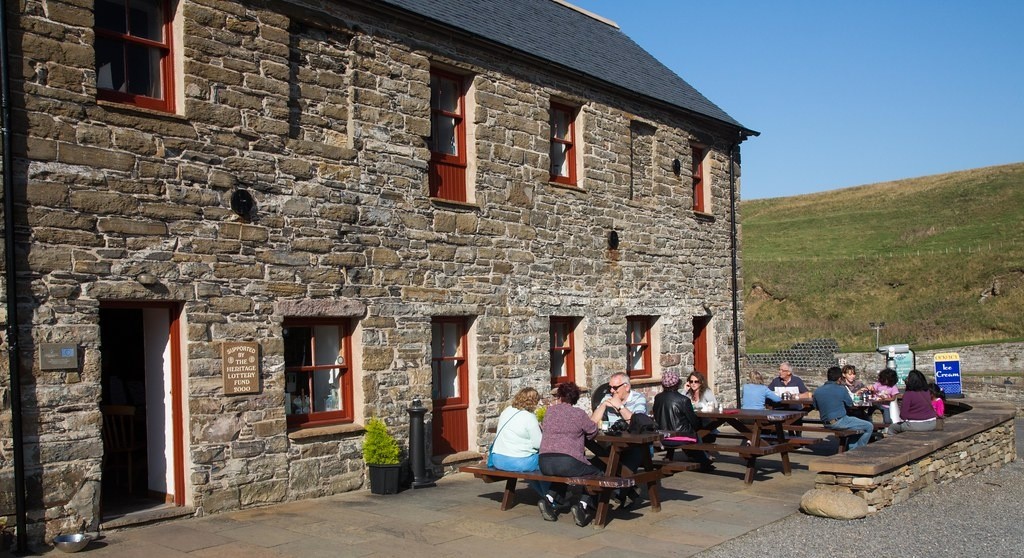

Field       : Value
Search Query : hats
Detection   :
[661,371,679,387]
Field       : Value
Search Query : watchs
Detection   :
[617,405,625,411]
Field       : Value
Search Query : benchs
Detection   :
[460,418,890,490]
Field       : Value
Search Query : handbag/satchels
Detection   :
[936,416,945,430]
[487,443,494,468]
[629,410,659,434]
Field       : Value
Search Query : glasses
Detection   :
[608,382,627,390]
[689,380,699,384]
[844,366,855,374]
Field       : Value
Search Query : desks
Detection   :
[768,397,890,454]
[650,408,803,486]
[483,429,664,528]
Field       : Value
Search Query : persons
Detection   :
[868,368,900,424]
[887,369,937,435]
[590,372,654,510]
[928,383,946,418]
[491,387,573,509]
[766,362,809,445]
[840,365,875,443]
[740,371,783,447]
[538,381,605,528]
[653,371,717,472]
[812,367,874,450]
[681,372,718,461]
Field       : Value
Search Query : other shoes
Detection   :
[614,483,641,509]
[571,503,586,526]
[537,498,557,521]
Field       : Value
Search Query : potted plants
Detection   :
[358,415,408,495]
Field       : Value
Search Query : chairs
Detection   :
[100,406,139,495]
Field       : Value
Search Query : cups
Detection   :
[601,421,609,431]
[782,393,788,400]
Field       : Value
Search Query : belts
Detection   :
[823,417,841,425]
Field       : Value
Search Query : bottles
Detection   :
[718,404,723,413]
[862,392,867,402]
[867,391,872,399]
